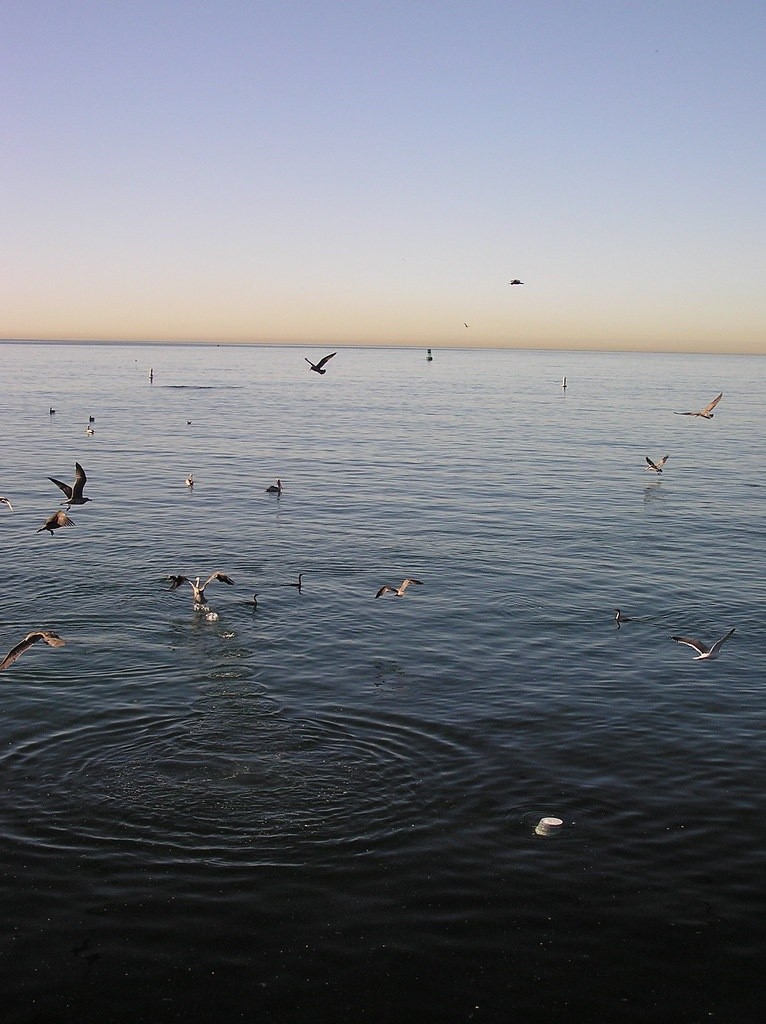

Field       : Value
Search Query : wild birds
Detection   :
[510,279,523,286]
[614,609,632,622]
[266,479,282,492]
[674,393,723,419]
[37,462,92,536]
[426,349,433,361]
[166,571,304,611]
[305,352,337,375]
[0,496,13,512]
[562,376,567,387]
[149,369,154,378]
[0,631,66,672]
[669,628,735,660]
[186,474,194,486]
[185,421,192,425]
[464,322,469,327]
[50,406,95,435]
[644,456,668,475]
[375,579,423,599]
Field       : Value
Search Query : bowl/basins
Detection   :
[535,818,563,836]
